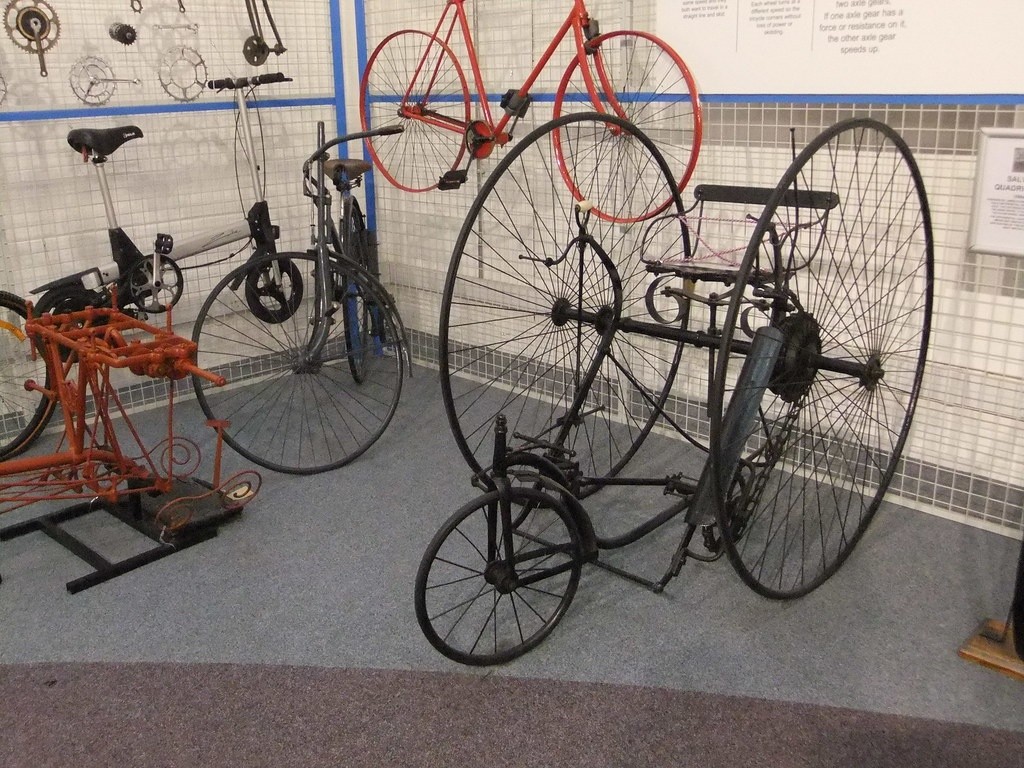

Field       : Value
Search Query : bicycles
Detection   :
[1,293,57,465]
[359,0,702,226]
[189,124,409,477]
[25,67,296,367]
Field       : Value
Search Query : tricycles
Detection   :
[412,111,933,671]
